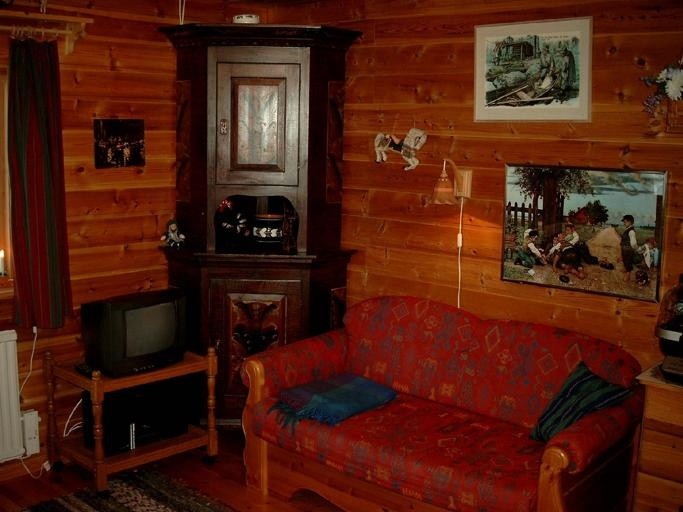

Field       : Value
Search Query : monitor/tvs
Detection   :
[80,286,188,377]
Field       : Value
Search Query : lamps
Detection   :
[424,153,475,206]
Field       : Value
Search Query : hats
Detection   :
[621,215,633,221]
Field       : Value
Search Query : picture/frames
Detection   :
[474,15,594,125]
[498,160,668,306]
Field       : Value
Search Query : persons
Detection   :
[511,214,660,282]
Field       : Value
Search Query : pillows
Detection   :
[526,358,635,447]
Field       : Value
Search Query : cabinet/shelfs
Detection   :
[159,25,368,449]
[36,343,224,493]
[623,360,681,511]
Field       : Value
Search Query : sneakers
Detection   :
[514,258,522,265]
[624,275,630,281]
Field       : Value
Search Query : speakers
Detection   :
[82,383,162,457]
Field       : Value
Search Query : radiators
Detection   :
[1,330,29,466]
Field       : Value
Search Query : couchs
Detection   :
[238,290,641,512]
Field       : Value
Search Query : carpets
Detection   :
[21,465,238,512]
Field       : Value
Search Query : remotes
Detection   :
[75,362,102,377]
